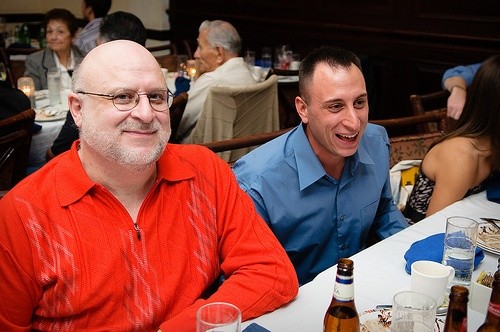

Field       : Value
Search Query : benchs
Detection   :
[206,114,455,168]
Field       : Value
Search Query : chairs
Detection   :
[0,41,177,88]
[410,92,448,134]
[266,66,303,130]
[0,109,36,191]
[170,92,188,143]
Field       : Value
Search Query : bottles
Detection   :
[13,26,20,47]
[323,258,361,332]
[443,286,469,332]
[23,26,31,47]
[40,28,46,49]
[477,271,500,332]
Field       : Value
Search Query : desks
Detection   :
[31,70,299,161]
[207,190,500,332]
[10,38,171,62]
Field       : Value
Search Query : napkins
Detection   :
[404,231,484,277]
[242,323,271,332]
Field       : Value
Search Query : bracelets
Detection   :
[450,85,466,93]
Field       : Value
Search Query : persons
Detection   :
[23,0,147,159]
[176,20,257,138]
[0,87,33,191]
[232,50,410,284]
[404,58,500,225]
[442,63,480,120]
[0,40,298,332]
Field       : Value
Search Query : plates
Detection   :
[357,308,441,332]
[476,223,500,255]
[34,113,66,122]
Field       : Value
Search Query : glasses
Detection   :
[74,87,175,112]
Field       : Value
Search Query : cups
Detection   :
[260,47,272,68]
[410,260,455,305]
[390,292,438,332]
[244,51,254,66]
[442,216,478,286]
[195,302,242,332]
